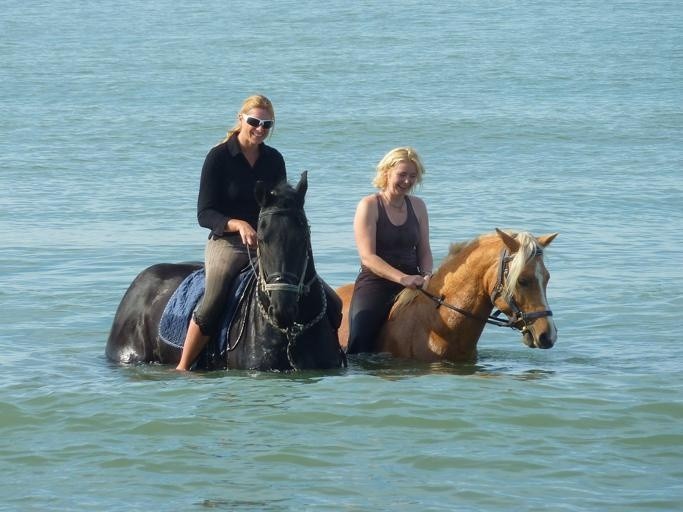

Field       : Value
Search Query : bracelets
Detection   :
[422,271,432,277]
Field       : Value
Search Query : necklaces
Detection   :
[383,192,404,212]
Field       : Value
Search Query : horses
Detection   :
[333,227,559,363]
[104,168,344,372]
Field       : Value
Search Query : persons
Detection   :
[343,145,433,366]
[176,94,345,372]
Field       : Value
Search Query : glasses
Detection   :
[243,114,273,129]
